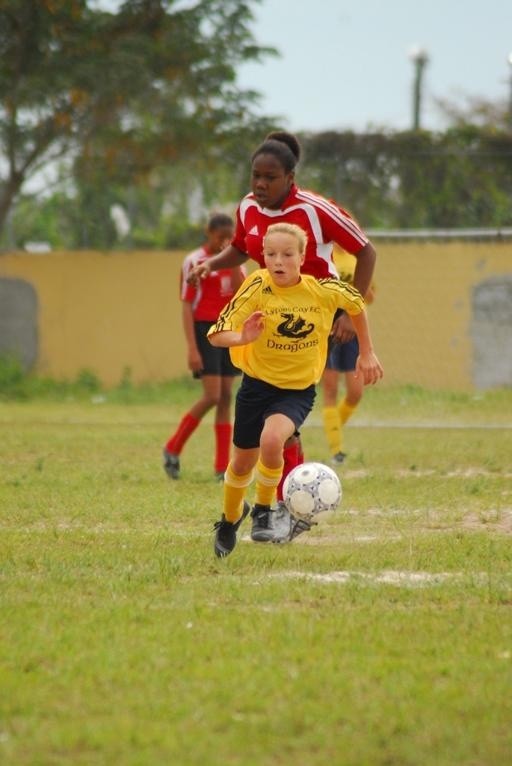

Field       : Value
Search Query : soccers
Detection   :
[281,462,344,524]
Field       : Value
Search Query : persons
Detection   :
[161,212,247,484]
[186,132,377,545]
[206,222,385,559]
[319,236,376,464]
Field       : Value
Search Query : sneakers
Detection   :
[214,500,250,558]
[163,449,180,480]
[250,504,311,544]
[332,453,345,464]
